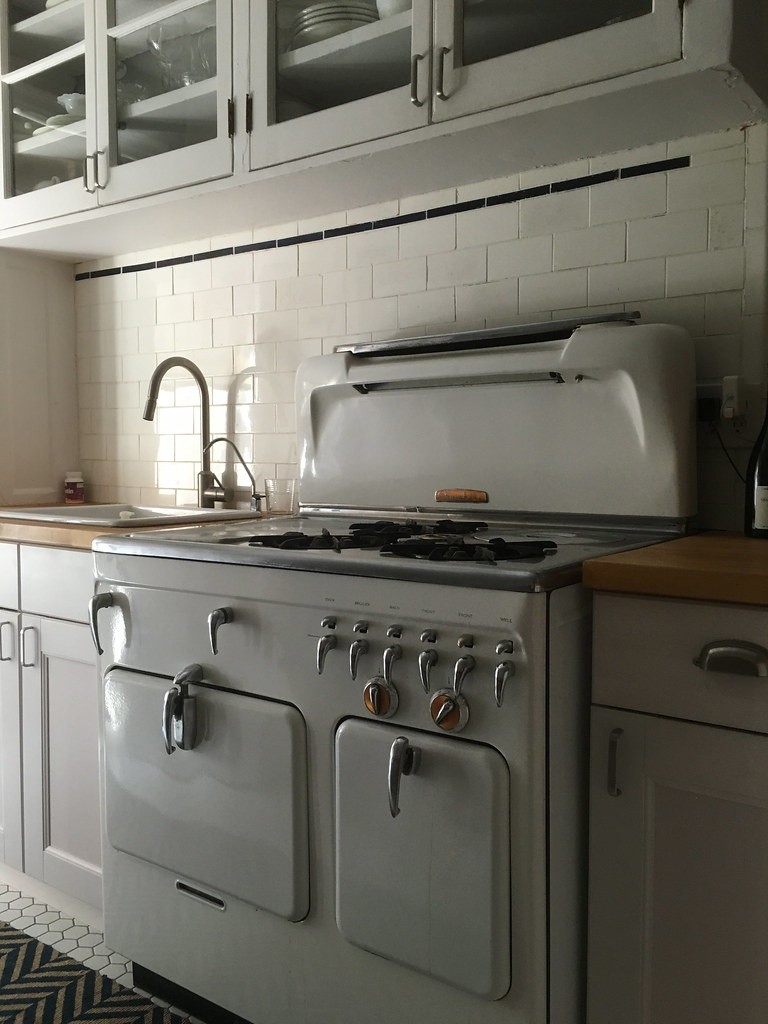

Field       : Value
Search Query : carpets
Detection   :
[0,919,193,1024]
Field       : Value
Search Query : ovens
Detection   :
[88,543,552,1023]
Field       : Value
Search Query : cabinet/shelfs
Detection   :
[0,541,104,912]
[0,0,235,265]
[245,1,768,230]
[585,588,767,1024]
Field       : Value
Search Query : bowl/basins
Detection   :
[57,92,85,119]
[376,0,412,21]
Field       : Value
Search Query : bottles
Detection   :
[744,398,768,539]
[65,472,85,503]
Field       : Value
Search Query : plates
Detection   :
[32,114,84,136]
[285,1,380,51]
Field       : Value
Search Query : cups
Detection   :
[114,58,149,110]
[264,478,294,515]
[176,25,216,89]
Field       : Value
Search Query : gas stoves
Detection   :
[126,312,696,583]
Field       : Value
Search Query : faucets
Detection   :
[202,438,267,511]
[144,357,233,508]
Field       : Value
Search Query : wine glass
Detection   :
[148,22,182,92]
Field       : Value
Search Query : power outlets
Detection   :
[695,384,762,448]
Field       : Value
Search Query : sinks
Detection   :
[0,503,264,528]
